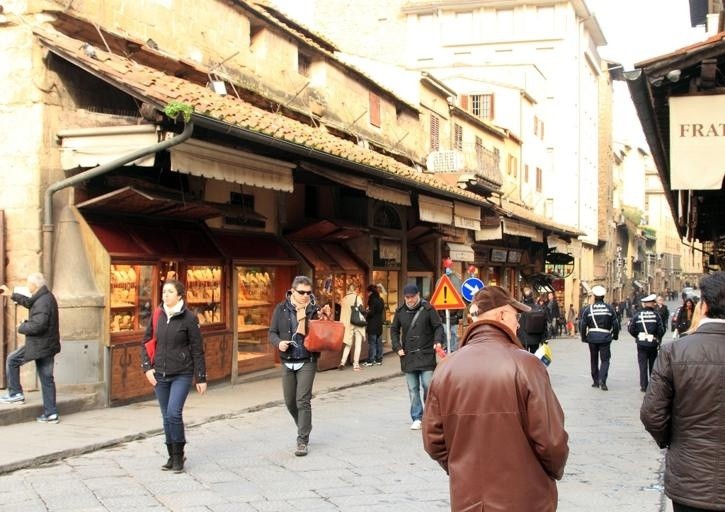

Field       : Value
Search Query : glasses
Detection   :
[294,288,312,295]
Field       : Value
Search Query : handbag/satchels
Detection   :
[305,306,344,352]
[145,338,158,366]
[350,306,369,326]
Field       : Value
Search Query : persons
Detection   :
[268,276,332,457]
[637,268,725,512]
[581,286,620,391]
[628,294,666,392]
[142,278,207,473]
[437,282,701,362]
[339,284,366,370]
[420,285,570,512]
[365,284,384,365]
[0,273,61,425]
[390,283,445,431]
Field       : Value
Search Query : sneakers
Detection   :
[639,386,646,392]
[410,420,422,430]
[0,392,26,406]
[339,361,382,371]
[36,413,60,424]
[295,443,308,456]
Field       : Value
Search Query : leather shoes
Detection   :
[591,382,608,392]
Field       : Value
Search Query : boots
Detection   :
[160,442,187,474]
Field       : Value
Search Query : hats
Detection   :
[404,284,419,296]
[592,286,606,296]
[640,294,657,302]
[469,286,531,316]
